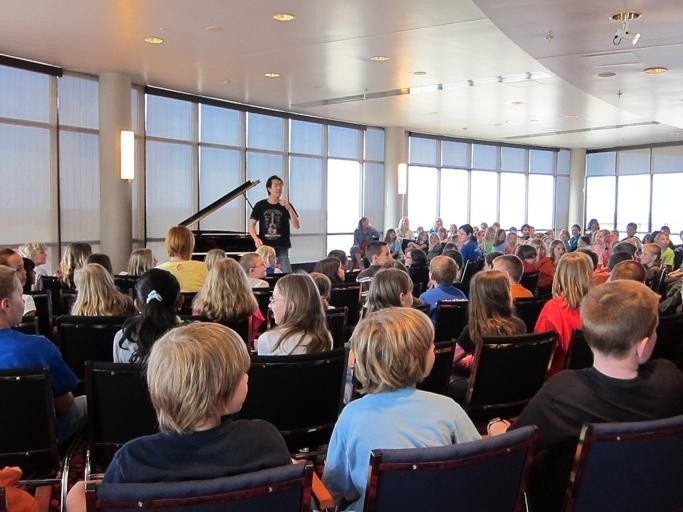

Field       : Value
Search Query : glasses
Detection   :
[268,296,285,303]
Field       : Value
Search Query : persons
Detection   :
[534,251,595,380]
[113,268,186,364]
[258,273,333,355]
[451,269,528,389]
[249,176,299,273]
[488,280,683,512]
[66,321,293,512]
[0,217,683,342]
[0,264,88,428]
[321,306,483,512]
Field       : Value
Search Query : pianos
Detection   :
[178,179,260,262]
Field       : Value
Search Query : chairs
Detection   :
[0,239,683,512]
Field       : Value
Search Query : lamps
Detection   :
[612,14,642,47]
[120,129,136,182]
